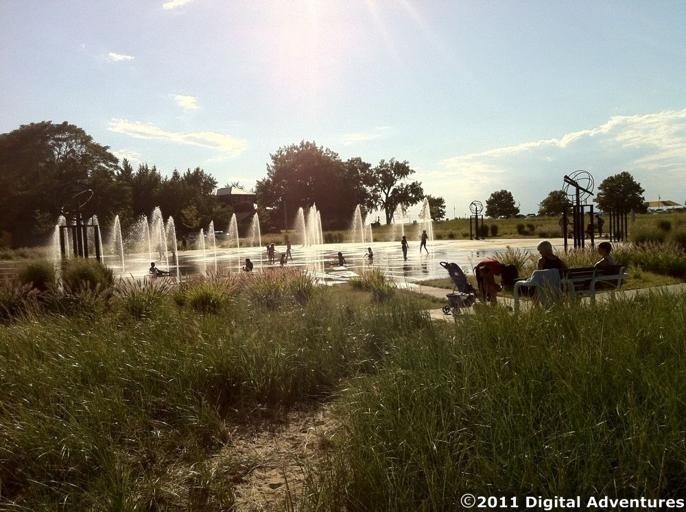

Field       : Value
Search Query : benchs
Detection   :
[513,265,629,310]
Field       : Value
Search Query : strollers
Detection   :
[440,261,478,315]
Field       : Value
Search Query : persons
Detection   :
[513,240,566,295]
[558,212,569,237]
[149,262,170,277]
[280,253,285,264]
[181,235,186,251]
[242,258,253,272]
[401,235,409,260]
[592,241,617,268]
[363,247,373,264]
[268,243,274,263]
[419,230,430,254]
[286,241,293,260]
[475,256,519,303]
[337,251,346,266]
[596,215,605,237]
